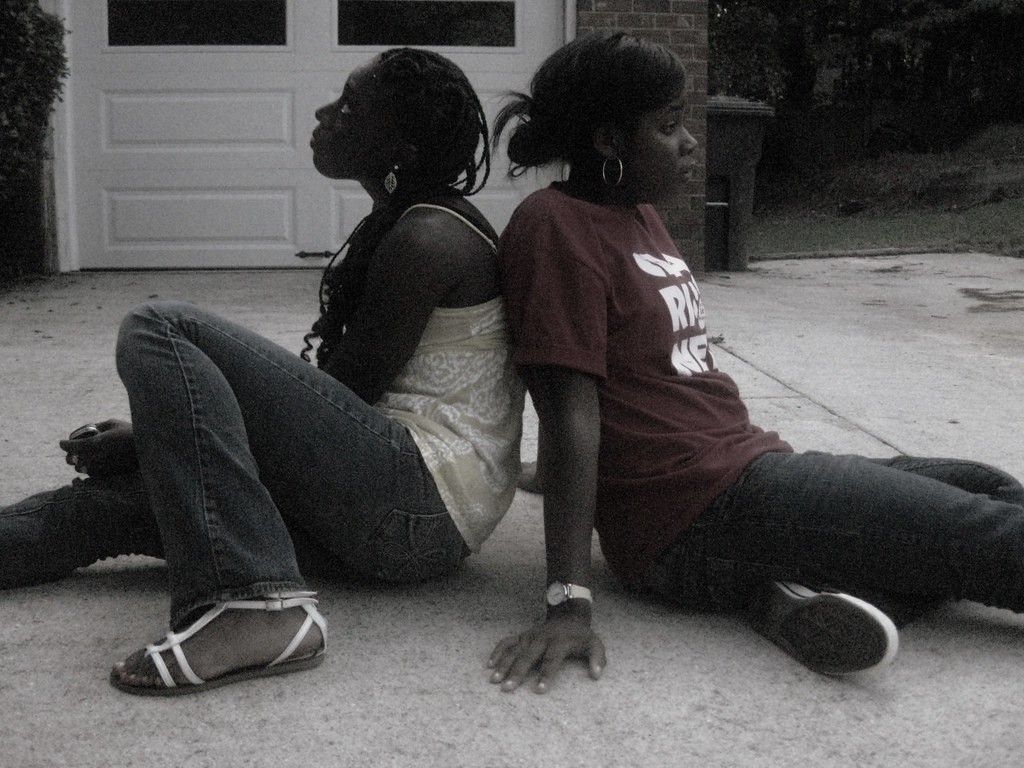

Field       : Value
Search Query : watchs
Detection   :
[545,581,592,606]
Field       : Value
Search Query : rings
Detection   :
[72,453,78,465]
[80,466,88,474]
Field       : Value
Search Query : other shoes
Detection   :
[740,579,898,678]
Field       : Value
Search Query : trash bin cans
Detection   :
[706,94,777,273]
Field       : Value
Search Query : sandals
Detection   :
[111,592,328,697]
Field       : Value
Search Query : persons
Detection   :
[0,47,528,696]
[486,25,1024,694]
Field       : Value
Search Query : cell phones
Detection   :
[70,423,101,441]
